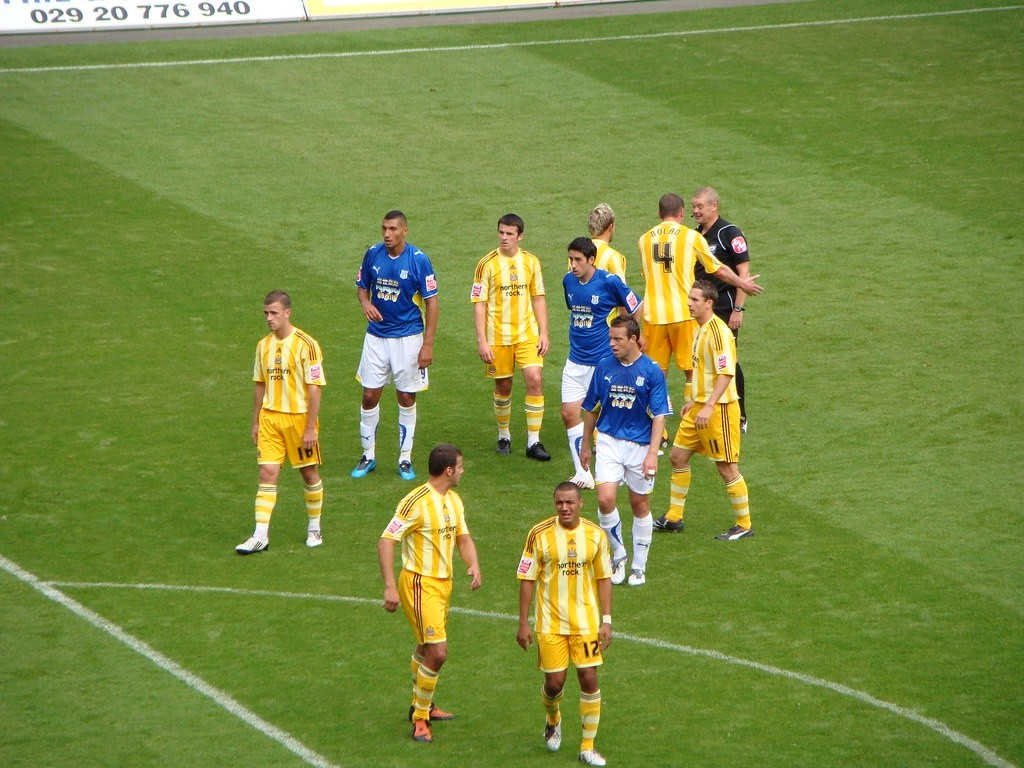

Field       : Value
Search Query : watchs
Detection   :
[733,305,745,312]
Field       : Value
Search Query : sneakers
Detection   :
[610,552,628,584]
[497,437,511,456]
[409,701,453,721]
[579,750,606,766]
[568,476,595,490]
[306,528,323,548]
[352,455,375,478]
[236,537,268,553]
[413,718,432,742]
[628,569,645,585]
[526,444,551,461]
[545,713,562,752]
[398,460,416,480]
[653,515,683,533]
[715,527,753,541]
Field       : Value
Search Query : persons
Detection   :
[234,290,326,554]
[689,187,751,435]
[559,236,643,492]
[515,481,613,765]
[377,444,482,742]
[637,191,763,447]
[579,314,674,585]
[350,210,438,479]
[566,201,628,327]
[652,279,756,539]
[471,212,554,460]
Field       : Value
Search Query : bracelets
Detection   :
[602,614,612,624]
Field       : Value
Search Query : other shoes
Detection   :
[740,421,749,433]
[660,437,670,448]
